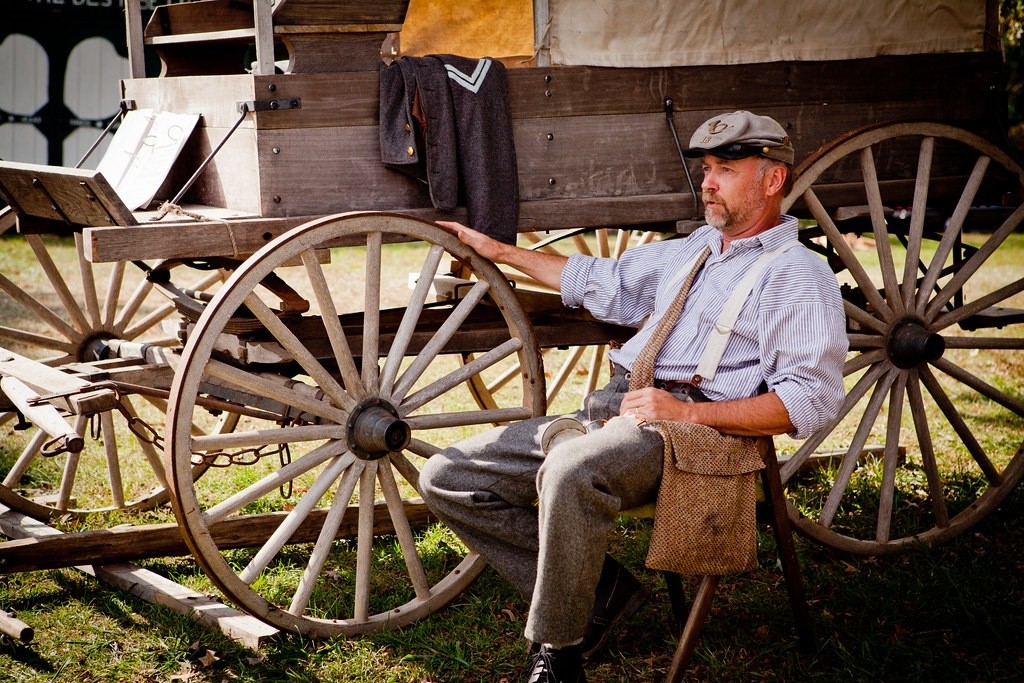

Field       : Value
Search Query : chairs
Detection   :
[617,436,816,683]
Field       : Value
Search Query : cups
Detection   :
[541,417,607,457]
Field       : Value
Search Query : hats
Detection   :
[681,110,794,166]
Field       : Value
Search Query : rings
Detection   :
[636,408,639,414]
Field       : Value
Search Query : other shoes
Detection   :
[581,564,649,657]
[526,638,586,683]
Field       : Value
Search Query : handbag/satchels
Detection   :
[645,419,766,575]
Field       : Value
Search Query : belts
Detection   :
[620,370,712,402]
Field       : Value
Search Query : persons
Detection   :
[415,110,845,683]
